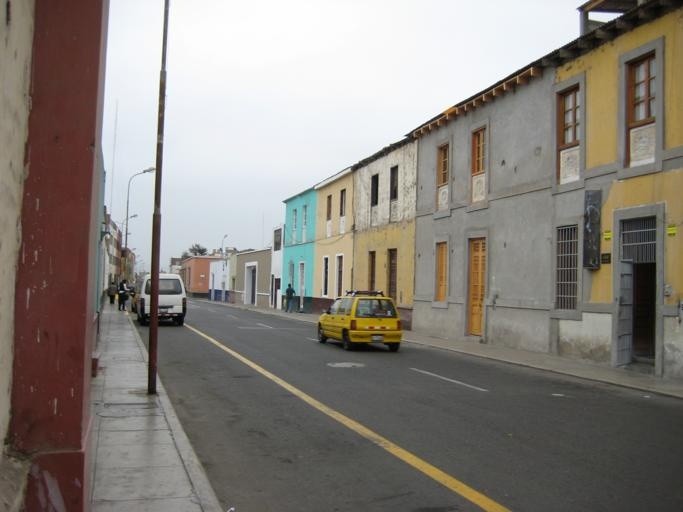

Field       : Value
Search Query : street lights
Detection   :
[120,165,156,280]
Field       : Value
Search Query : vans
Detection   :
[131,274,187,327]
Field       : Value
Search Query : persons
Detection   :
[284,283,296,314]
[106,282,116,304]
[117,279,133,311]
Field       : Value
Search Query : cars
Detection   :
[318,295,403,353]
